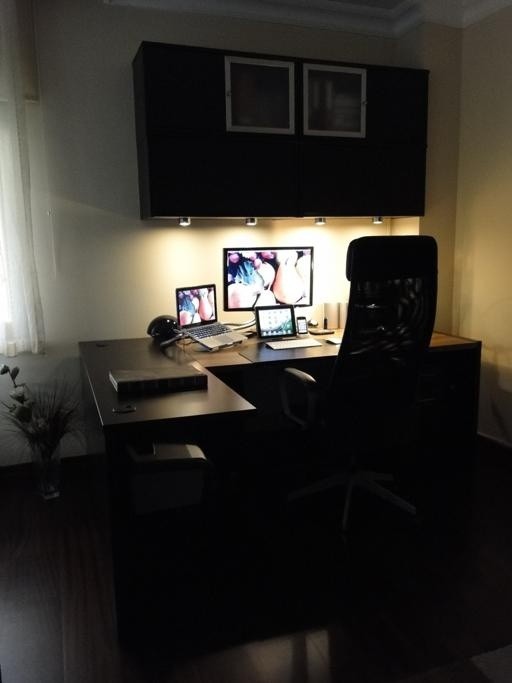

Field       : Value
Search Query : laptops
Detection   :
[176,284,248,349]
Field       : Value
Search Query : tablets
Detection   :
[255,305,297,340]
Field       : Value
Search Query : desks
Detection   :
[80,324,482,650]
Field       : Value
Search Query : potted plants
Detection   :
[2,366,93,502]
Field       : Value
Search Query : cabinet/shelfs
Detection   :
[130,37,430,220]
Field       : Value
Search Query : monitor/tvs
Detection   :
[223,246,314,311]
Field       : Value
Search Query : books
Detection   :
[110,362,208,392]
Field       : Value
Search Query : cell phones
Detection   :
[297,316,308,334]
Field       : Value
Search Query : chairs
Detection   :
[278,241,436,546]
